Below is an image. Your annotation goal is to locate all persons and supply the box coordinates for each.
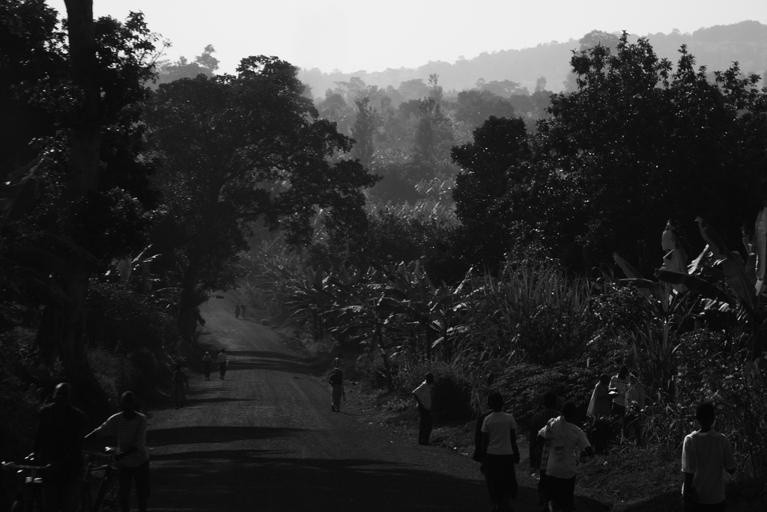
[35,382,153,511]
[327,365,346,413]
[200,348,229,381]
[474,391,520,511]
[680,401,738,512]
[172,364,189,409]
[530,400,595,512]
[587,365,645,455]
[410,370,436,447]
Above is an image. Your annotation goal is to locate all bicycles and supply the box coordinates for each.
[77,447,119,509]
[0,460,51,511]
